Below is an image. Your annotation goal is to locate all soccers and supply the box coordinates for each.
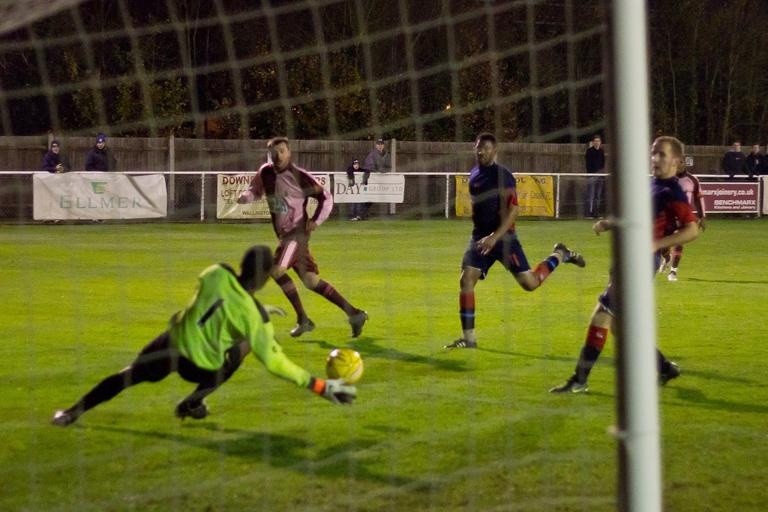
[326,349,363,384]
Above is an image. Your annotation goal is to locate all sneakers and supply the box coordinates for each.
[174,400,208,419]
[445,338,477,350]
[659,254,677,282]
[53,410,70,426]
[549,378,589,394]
[348,310,369,337]
[659,363,680,384]
[553,243,586,267]
[290,320,315,336]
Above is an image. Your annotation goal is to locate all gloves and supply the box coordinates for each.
[264,305,286,317]
[311,377,357,404]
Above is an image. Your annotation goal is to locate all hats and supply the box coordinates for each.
[96,135,106,143]
[376,138,385,144]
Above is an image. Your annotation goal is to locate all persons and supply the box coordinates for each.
[364,138,392,173]
[660,156,706,283]
[746,143,765,176]
[585,135,605,220]
[50,244,358,425]
[346,159,370,221]
[765,145,768,175]
[235,137,369,338]
[42,141,66,224]
[722,139,753,220]
[442,132,585,351]
[85,133,117,225]
[547,136,707,394]
